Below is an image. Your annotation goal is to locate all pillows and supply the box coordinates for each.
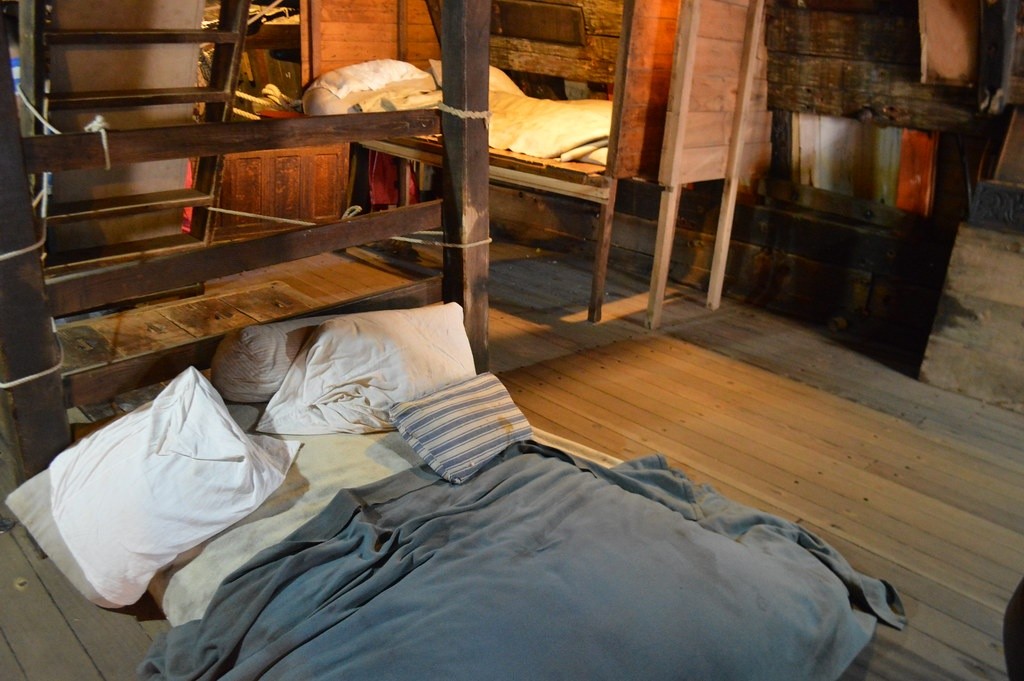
[427,58,526,97]
[252,302,477,435]
[3,364,304,612]
[305,58,429,100]
[299,87,442,119]
[386,372,534,484]
[211,301,444,403]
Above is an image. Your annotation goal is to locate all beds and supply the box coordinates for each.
[343,102,735,326]
[60,374,910,679]
